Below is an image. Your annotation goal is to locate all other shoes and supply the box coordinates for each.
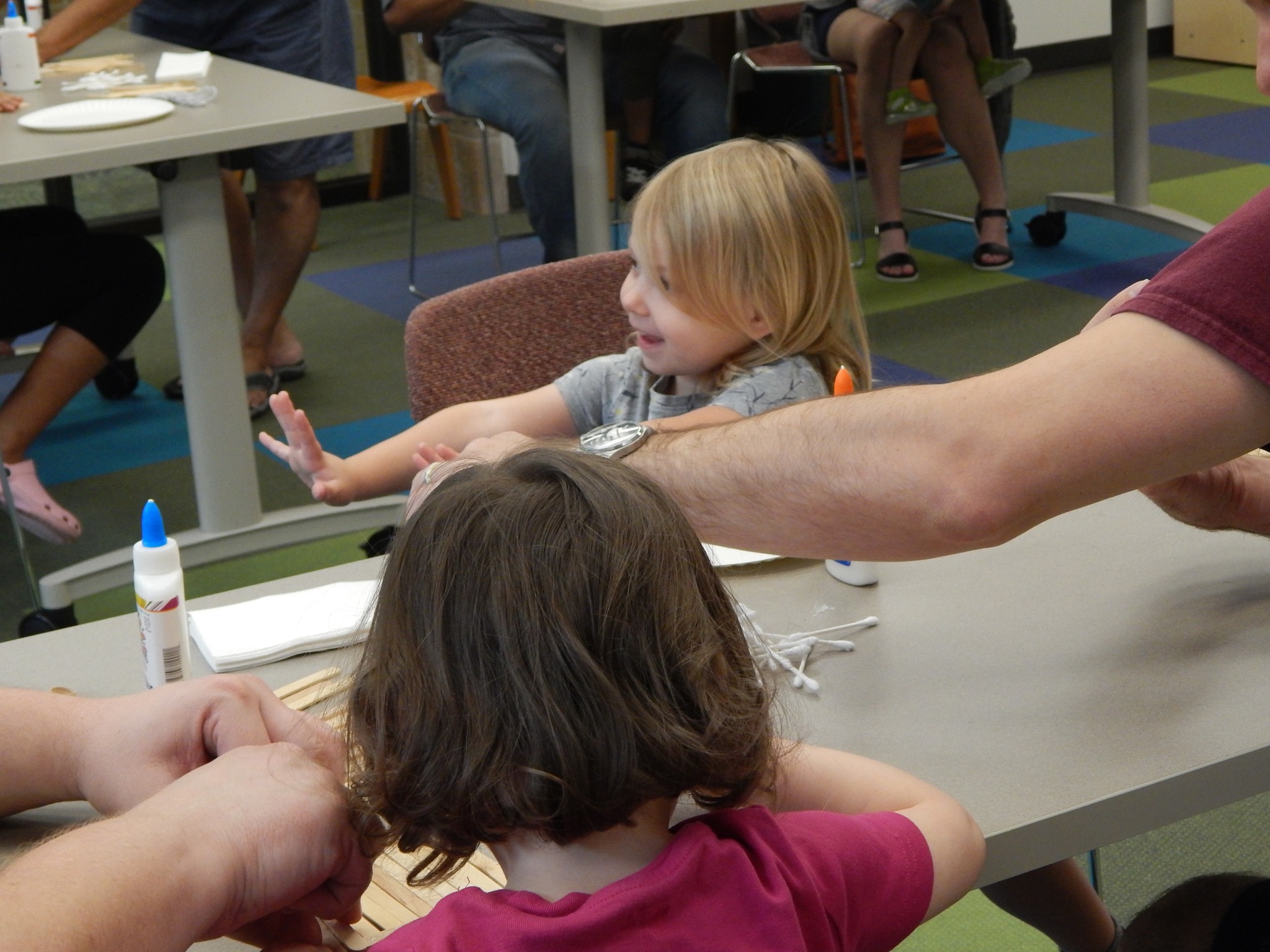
[885,87,939,125]
[978,56,1032,99]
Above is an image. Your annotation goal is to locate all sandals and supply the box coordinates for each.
[971,199,1015,272]
[871,215,920,282]
[0,458,82,545]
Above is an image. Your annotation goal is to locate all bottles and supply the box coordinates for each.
[0,0,43,93]
[133,498,187,691]
[824,364,879,585]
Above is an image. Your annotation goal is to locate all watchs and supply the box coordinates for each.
[577,421,659,462]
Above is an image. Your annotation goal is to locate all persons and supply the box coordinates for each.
[0,0,1031,541]
[338,451,986,952]
[258,139,1129,952]
[0,669,385,952]
[400,0,1270,952]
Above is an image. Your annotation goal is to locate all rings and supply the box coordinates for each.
[426,462,438,483]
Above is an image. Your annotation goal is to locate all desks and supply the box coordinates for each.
[2,488,1269,952]
[0,17,411,639]
[477,1,1219,268]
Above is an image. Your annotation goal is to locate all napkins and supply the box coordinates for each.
[154,51,213,82]
[188,579,384,672]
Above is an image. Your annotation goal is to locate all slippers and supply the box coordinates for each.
[244,370,281,422]
[272,359,307,381]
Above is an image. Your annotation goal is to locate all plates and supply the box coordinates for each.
[16,97,175,132]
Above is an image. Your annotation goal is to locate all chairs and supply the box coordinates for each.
[408,33,667,289]
[403,248,1101,896]
[726,0,1012,268]
